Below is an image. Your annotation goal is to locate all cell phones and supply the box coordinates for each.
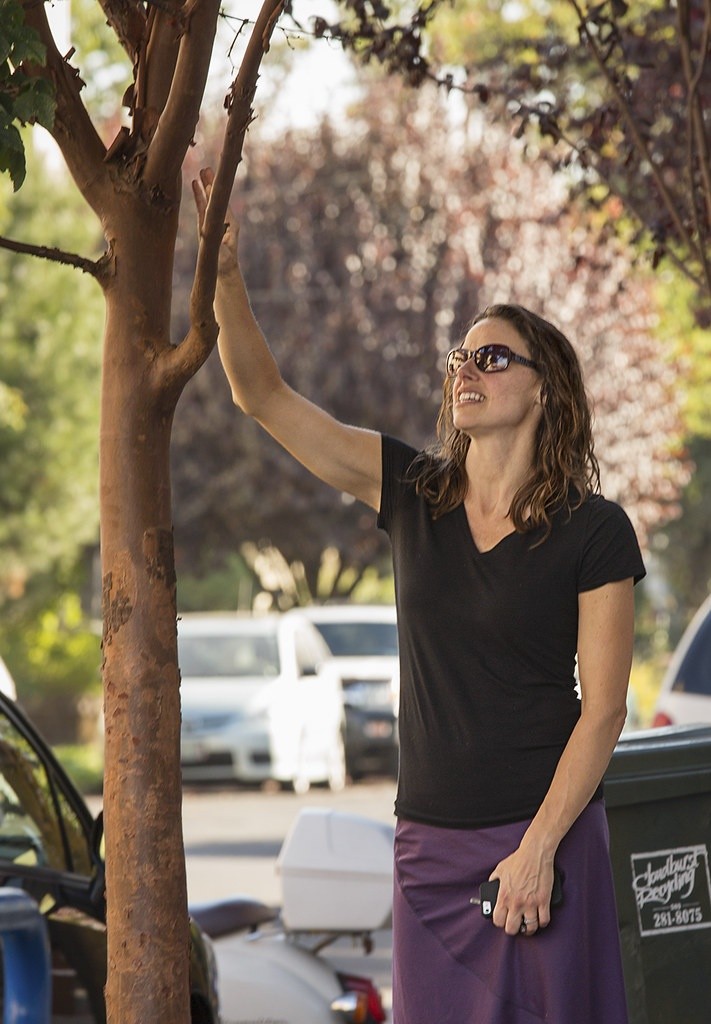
[479,873,565,916]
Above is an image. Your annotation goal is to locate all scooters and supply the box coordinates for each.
[186,801,395,1024]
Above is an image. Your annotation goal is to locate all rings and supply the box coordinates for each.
[524,918,538,924]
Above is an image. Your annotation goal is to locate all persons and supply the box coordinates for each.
[191,168,646,1024]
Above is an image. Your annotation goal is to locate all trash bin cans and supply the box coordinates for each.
[602,724,711,1023]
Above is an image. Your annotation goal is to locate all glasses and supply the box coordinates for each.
[446,343,547,378]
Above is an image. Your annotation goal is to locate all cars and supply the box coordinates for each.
[2,686,223,1024]
[94,610,349,795]
[651,590,711,732]
[295,602,402,785]
